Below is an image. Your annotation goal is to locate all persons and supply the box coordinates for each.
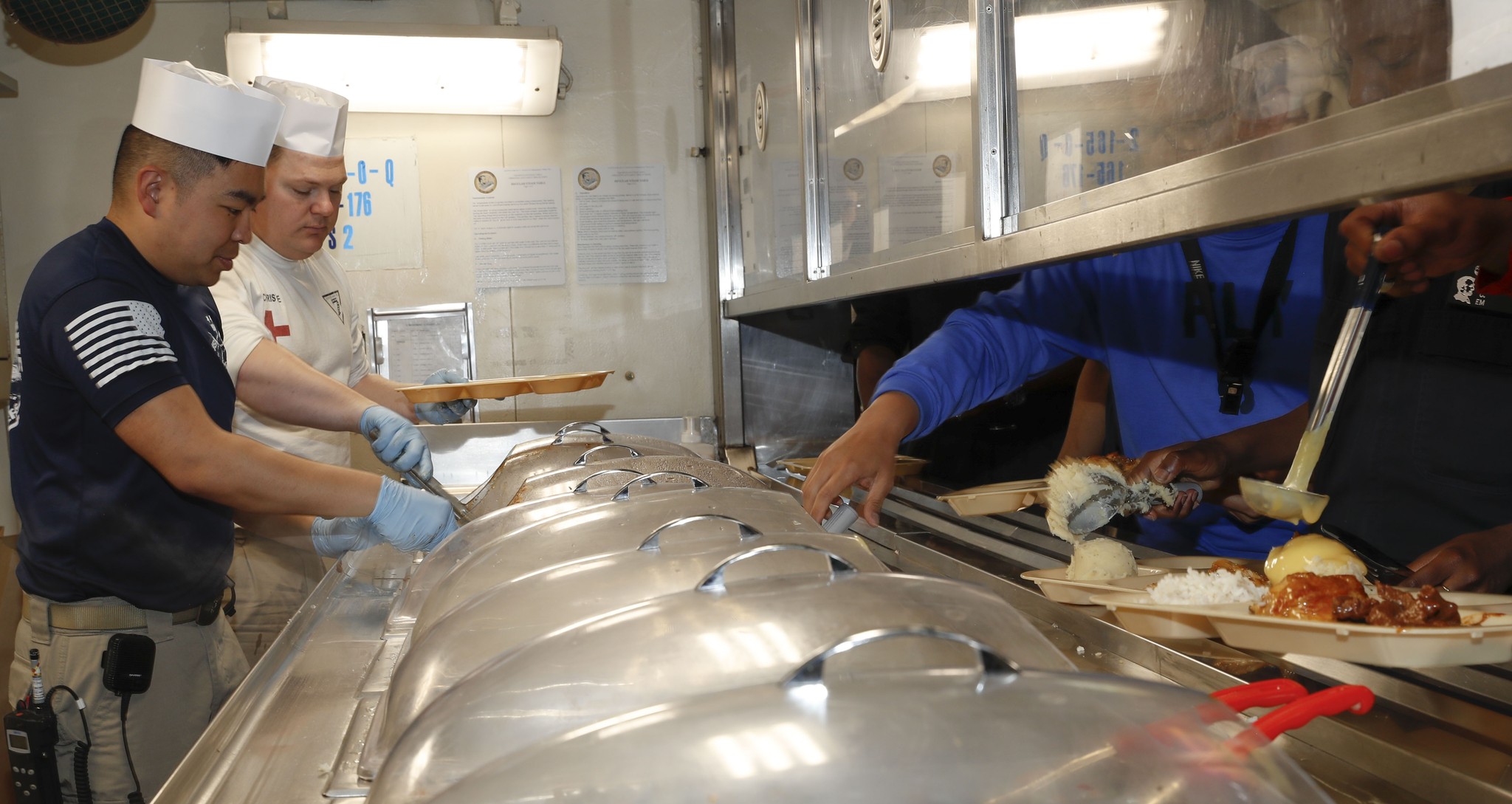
[5,57,461,804]
[208,76,504,672]
[800,0,1512,726]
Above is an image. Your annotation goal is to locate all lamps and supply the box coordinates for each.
[222,0,566,119]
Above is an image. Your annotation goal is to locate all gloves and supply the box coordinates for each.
[365,475,460,555]
[312,517,385,559]
[360,405,434,489]
[417,369,479,426]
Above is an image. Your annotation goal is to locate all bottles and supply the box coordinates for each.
[681,416,701,443]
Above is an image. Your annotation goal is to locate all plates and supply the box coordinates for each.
[394,370,616,405]
[937,479,1049,517]
[779,453,931,480]
[1020,556,1267,606]
[1089,585,1512,669]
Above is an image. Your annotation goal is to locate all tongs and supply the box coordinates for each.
[369,428,470,519]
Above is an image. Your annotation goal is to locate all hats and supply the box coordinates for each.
[253,75,349,158]
[131,57,286,167]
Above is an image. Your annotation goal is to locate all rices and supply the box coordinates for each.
[1145,567,1271,606]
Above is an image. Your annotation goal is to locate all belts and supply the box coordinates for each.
[20,591,223,629]
